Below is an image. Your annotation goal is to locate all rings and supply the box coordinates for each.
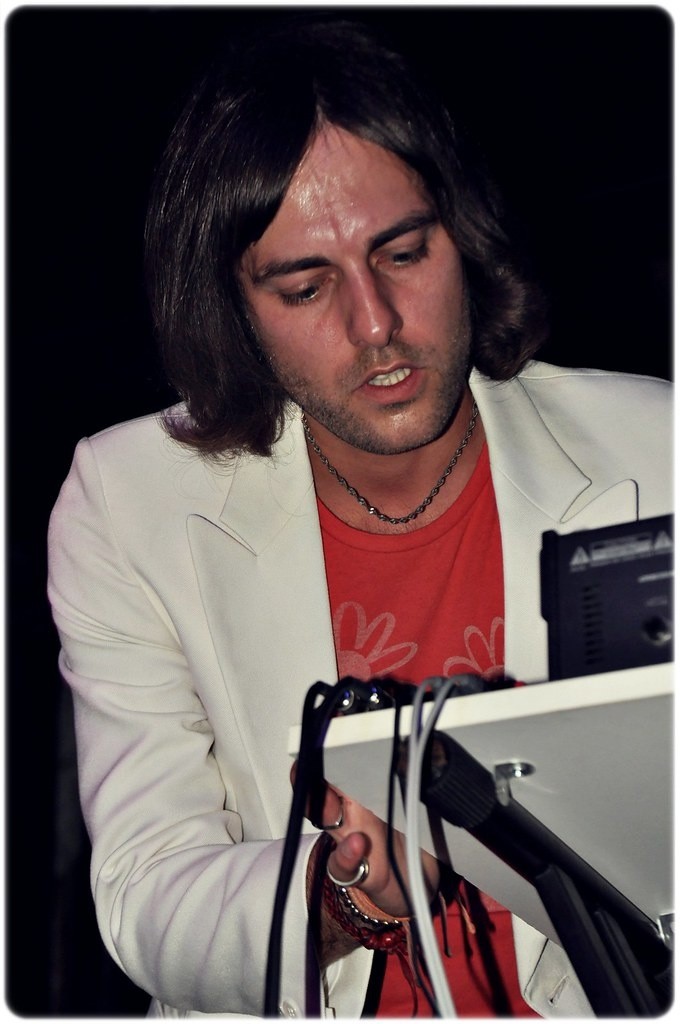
[310,805,344,831]
[326,857,370,888]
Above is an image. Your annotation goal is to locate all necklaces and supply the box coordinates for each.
[300,399,480,524]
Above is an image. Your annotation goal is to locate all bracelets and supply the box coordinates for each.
[321,837,476,1018]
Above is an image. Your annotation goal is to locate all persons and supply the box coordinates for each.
[48,25,675,1019]
